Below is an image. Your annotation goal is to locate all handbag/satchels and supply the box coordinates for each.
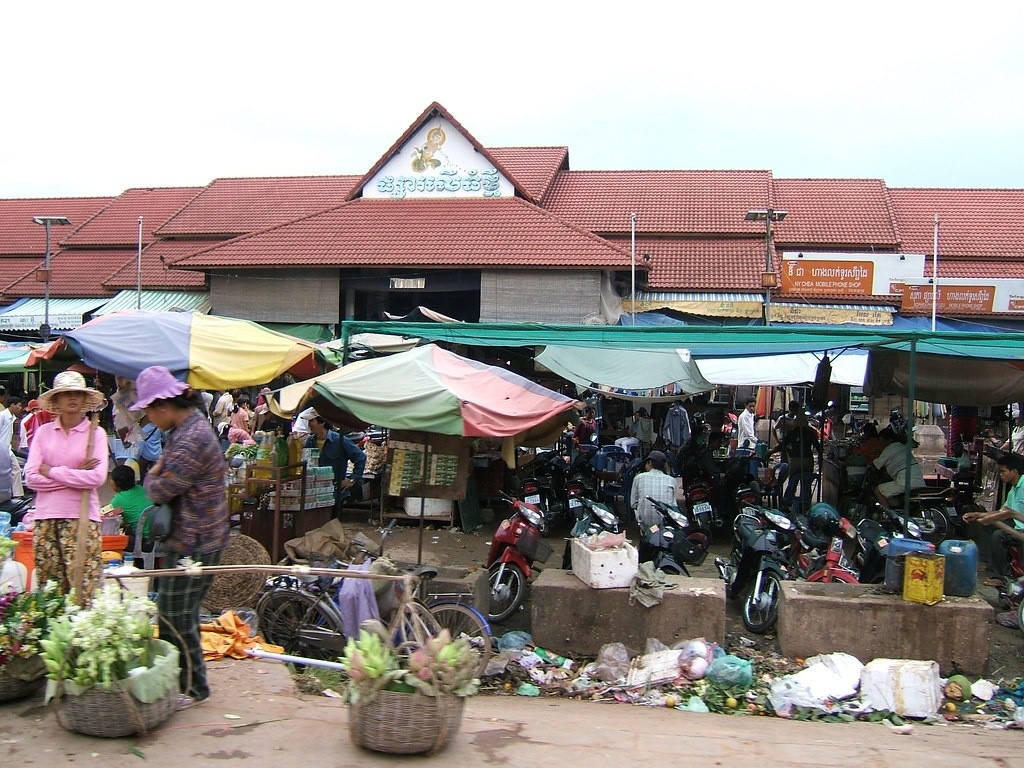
[148,502,172,540]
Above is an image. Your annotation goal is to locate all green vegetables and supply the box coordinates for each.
[223,443,260,459]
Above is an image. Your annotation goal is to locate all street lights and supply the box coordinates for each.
[742,209,789,328]
[32,215,73,342]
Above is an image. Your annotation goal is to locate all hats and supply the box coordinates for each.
[640,451,667,462]
[25,400,38,412]
[300,407,319,420]
[218,422,229,436]
[38,371,104,414]
[261,387,271,395]
[794,415,809,426]
[258,403,269,414]
[129,366,190,413]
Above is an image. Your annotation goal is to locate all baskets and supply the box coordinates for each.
[200,533,272,614]
[0,676,45,704]
[56,638,179,737]
[672,532,709,566]
[517,528,553,564]
[349,693,465,754]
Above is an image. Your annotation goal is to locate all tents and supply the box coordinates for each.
[340,319,1024,539]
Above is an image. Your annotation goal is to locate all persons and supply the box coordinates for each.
[630,451,677,532]
[772,400,800,483]
[628,411,644,437]
[110,465,155,552]
[572,406,597,454]
[0,387,40,505]
[128,364,229,712]
[25,370,109,609]
[769,412,821,513]
[737,396,764,450]
[852,421,883,460]
[199,387,279,445]
[870,428,927,508]
[962,455,1024,586]
[139,423,167,486]
[676,425,725,479]
[1000,410,1024,455]
[604,402,621,429]
[292,406,367,521]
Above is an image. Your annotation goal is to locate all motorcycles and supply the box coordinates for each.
[567,450,604,520]
[640,495,715,578]
[783,500,865,584]
[858,500,920,584]
[847,462,955,546]
[720,409,739,438]
[504,447,570,535]
[488,487,555,622]
[654,409,724,540]
[805,399,838,452]
[690,410,713,450]
[843,413,881,440]
[0,497,35,527]
[344,431,389,450]
[887,406,910,444]
[722,437,765,511]
[562,490,624,567]
[715,480,808,633]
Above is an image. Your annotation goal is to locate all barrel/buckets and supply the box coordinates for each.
[885,537,980,606]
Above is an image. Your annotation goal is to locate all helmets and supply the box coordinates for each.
[805,502,842,538]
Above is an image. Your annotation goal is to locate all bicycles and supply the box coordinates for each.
[257,521,492,689]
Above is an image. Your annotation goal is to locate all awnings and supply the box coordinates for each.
[0,290,210,331]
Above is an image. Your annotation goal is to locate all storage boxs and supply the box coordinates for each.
[570,538,638,590]
[402,495,452,518]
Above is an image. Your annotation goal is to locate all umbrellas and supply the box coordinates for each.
[25,306,344,391]
[0,341,43,373]
[256,342,587,565]
[142,610,351,675]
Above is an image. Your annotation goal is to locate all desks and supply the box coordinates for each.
[934,463,959,488]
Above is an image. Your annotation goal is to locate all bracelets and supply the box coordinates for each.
[349,479,354,483]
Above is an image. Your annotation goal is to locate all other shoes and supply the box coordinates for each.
[979,561,989,572]
[983,577,1004,586]
[995,610,1020,628]
[179,694,210,710]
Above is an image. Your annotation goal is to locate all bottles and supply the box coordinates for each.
[1,556,151,606]
[253,428,303,483]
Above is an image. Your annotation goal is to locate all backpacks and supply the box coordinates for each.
[682,449,710,481]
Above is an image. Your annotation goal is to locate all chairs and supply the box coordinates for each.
[575,442,642,520]
[124,504,164,592]
